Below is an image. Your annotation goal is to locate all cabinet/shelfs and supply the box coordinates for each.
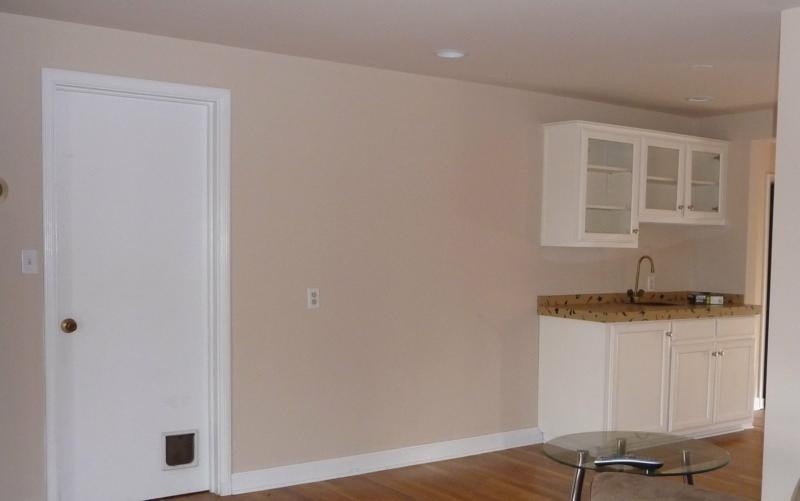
[641,129,726,226]
[670,316,756,435]
[538,119,642,249]
[537,317,671,453]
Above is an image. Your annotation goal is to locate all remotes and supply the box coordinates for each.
[594,455,665,470]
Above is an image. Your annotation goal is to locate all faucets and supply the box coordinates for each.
[635,254,654,296]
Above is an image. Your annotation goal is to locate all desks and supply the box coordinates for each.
[540,430,733,500]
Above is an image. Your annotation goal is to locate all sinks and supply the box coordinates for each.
[631,303,677,306]
